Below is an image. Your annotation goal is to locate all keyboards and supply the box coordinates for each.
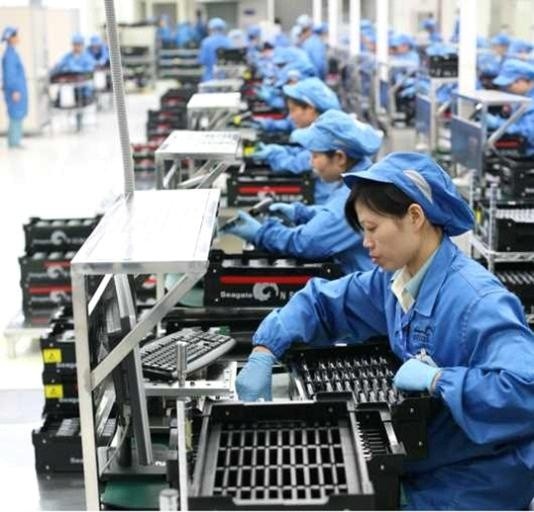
[133,328,236,382]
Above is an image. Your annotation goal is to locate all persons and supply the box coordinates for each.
[156,13,173,47]
[0,25,30,149]
[449,8,463,45]
[476,53,500,89]
[213,106,385,274]
[290,11,311,46]
[477,37,488,50]
[418,12,444,56]
[360,18,374,96]
[191,9,208,46]
[195,18,235,81]
[247,75,339,134]
[241,22,264,61]
[387,24,430,120]
[175,20,191,49]
[87,35,110,69]
[256,40,318,81]
[489,35,510,54]
[292,25,326,81]
[234,148,534,507]
[484,59,534,159]
[229,27,247,45]
[48,33,97,133]
[509,40,534,59]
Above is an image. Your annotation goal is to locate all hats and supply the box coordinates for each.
[311,21,329,35]
[269,47,312,67]
[71,29,84,46]
[246,25,263,43]
[502,34,531,55]
[489,56,532,93]
[280,73,339,116]
[0,20,19,44]
[475,48,502,78]
[282,63,320,84]
[337,149,479,239]
[89,36,101,48]
[384,30,415,54]
[205,15,226,34]
[287,107,388,161]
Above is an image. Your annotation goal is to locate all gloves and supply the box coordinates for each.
[389,356,444,398]
[219,212,263,249]
[252,141,277,163]
[232,350,277,409]
[266,199,297,229]
